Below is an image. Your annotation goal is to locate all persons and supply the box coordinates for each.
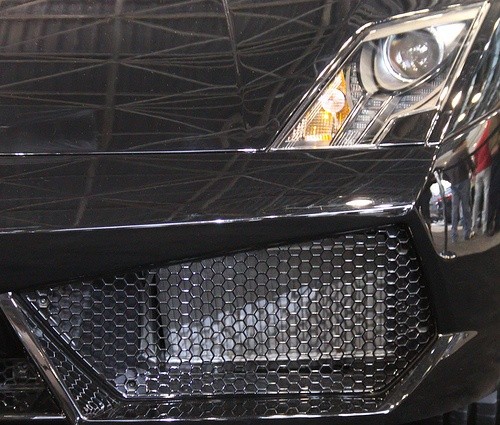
[443,138,476,244]
[471,132,499,236]
